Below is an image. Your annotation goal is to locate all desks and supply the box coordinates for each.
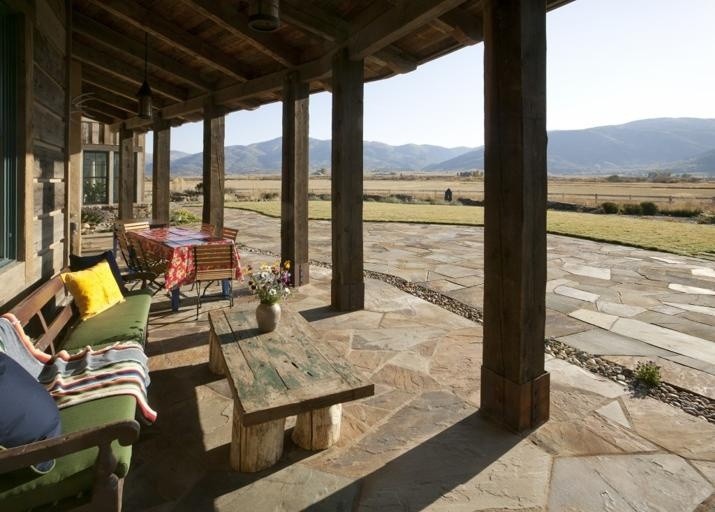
[207,305,375,474]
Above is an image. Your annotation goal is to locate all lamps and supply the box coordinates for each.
[136,32,153,119]
[247,0,281,34]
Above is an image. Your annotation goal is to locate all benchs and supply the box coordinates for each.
[0,266,157,512]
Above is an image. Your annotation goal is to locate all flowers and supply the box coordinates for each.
[243,256,297,305]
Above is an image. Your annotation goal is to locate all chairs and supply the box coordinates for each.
[114,220,239,320]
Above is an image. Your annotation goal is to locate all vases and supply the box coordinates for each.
[255,304,281,332]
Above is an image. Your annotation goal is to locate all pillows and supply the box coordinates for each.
[0,351,61,474]
[59,249,128,323]
